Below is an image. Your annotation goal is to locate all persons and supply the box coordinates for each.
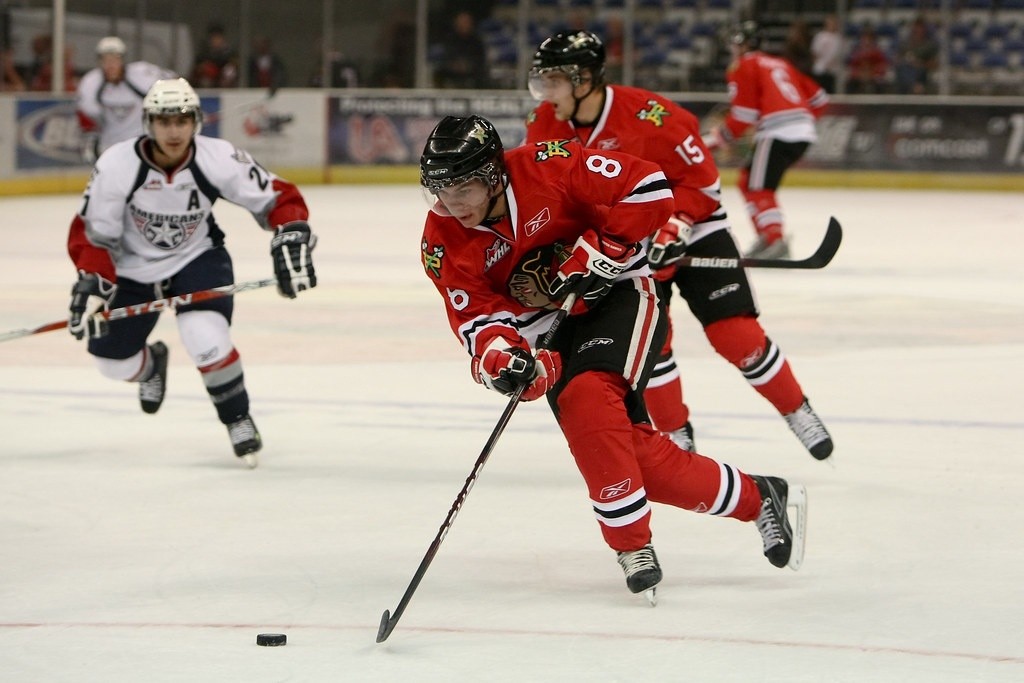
[0,34,77,91]
[186,26,294,88]
[636,4,722,117]
[526,30,833,461]
[700,20,830,258]
[603,18,641,85]
[67,77,318,457]
[311,37,346,88]
[787,15,939,95]
[432,9,486,89]
[421,113,794,596]
[74,36,180,166]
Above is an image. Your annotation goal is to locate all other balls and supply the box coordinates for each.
[256,633,287,647]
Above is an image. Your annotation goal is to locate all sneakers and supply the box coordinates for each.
[617,542,663,607]
[226,414,263,467]
[139,341,168,414]
[739,226,790,260]
[783,395,834,461]
[747,474,808,571]
[662,420,696,455]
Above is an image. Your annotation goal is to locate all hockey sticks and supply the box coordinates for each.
[674,216,843,270]
[0,277,279,342]
[375,291,576,644]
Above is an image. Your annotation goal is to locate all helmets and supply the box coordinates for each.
[95,36,127,55]
[728,21,764,52]
[143,77,200,116]
[419,115,505,193]
[527,28,607,85]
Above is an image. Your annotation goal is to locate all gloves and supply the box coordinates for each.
[80,131,99,165]
[648,222,679,282]
[68,267,118,341]
[471,325,562,402]
[270,221,317,299]
[547,227,637,316]
